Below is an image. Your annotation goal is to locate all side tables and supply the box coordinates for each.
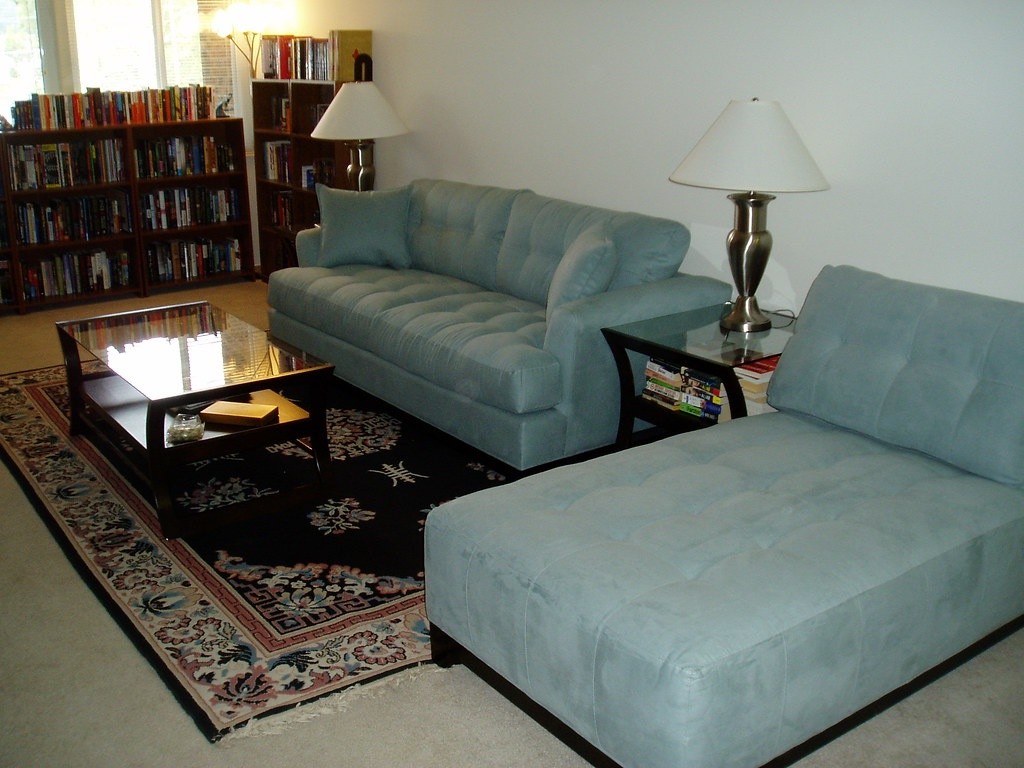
[601,305,797,455]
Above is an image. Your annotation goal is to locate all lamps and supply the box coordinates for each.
[211,0,298,79]
[668,100,832,331]
[309,82,406,190]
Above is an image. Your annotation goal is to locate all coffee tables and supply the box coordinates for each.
[54,301,335,542]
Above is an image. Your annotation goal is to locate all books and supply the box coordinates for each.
[185,393,245,414]
[4,83,246,303]
[63,303,231,354]
[199,400,279,428]
[262,94,332,231]
[639,354,782,424]
[260,29,373,83]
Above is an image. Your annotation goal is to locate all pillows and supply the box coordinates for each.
[546,219,616,326]
[315,183,409,270]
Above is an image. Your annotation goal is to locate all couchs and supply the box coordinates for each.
[268,177,732,478]
[423,264,1024,768]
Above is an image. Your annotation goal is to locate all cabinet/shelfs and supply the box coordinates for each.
[3,117,142,314]
[130,118,256,299]
[252,79,350,283]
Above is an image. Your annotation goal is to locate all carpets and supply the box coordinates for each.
[0,330,520,747]
